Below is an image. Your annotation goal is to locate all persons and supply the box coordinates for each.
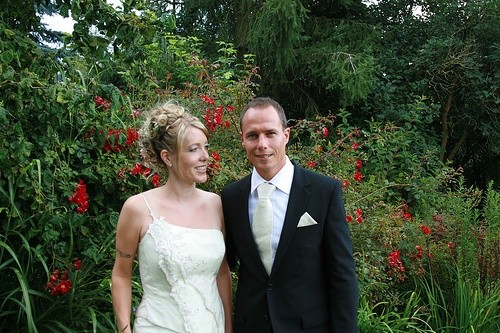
[218,98,359,332]
[111,100,233,333]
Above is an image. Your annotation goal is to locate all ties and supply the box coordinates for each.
[251,183,277,276]
